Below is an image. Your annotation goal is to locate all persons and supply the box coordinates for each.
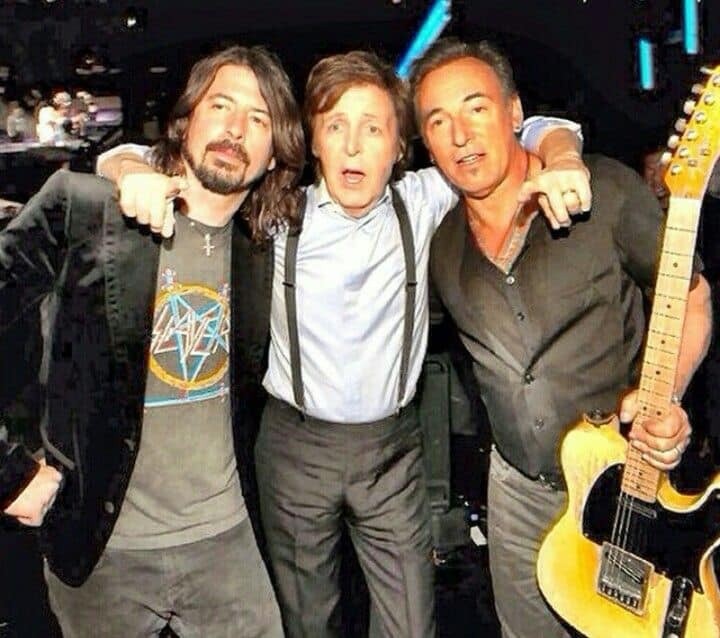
[0,42,720,638]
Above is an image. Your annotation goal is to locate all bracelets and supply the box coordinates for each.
[672,393,683,406]
[550,154,584,167]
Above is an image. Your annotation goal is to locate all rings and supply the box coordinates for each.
[562,189,576,196]
[675,446,681,457]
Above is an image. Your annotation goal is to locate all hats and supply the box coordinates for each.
[53,92,72,106]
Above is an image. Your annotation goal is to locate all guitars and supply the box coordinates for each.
[534,64,720,638]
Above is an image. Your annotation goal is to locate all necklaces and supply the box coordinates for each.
[493,147,531,266]
[177,210,234,258]
